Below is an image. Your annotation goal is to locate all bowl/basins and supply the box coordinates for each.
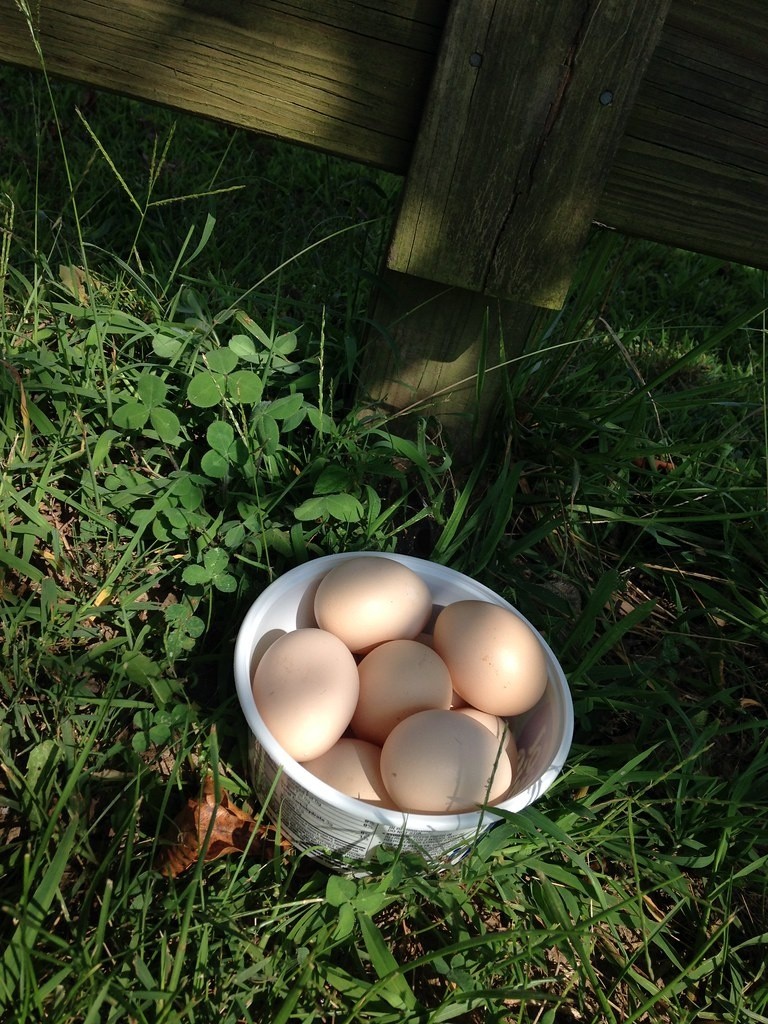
[235,550,576,874]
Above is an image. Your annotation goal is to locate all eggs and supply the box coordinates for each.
[251,556,548,814]
